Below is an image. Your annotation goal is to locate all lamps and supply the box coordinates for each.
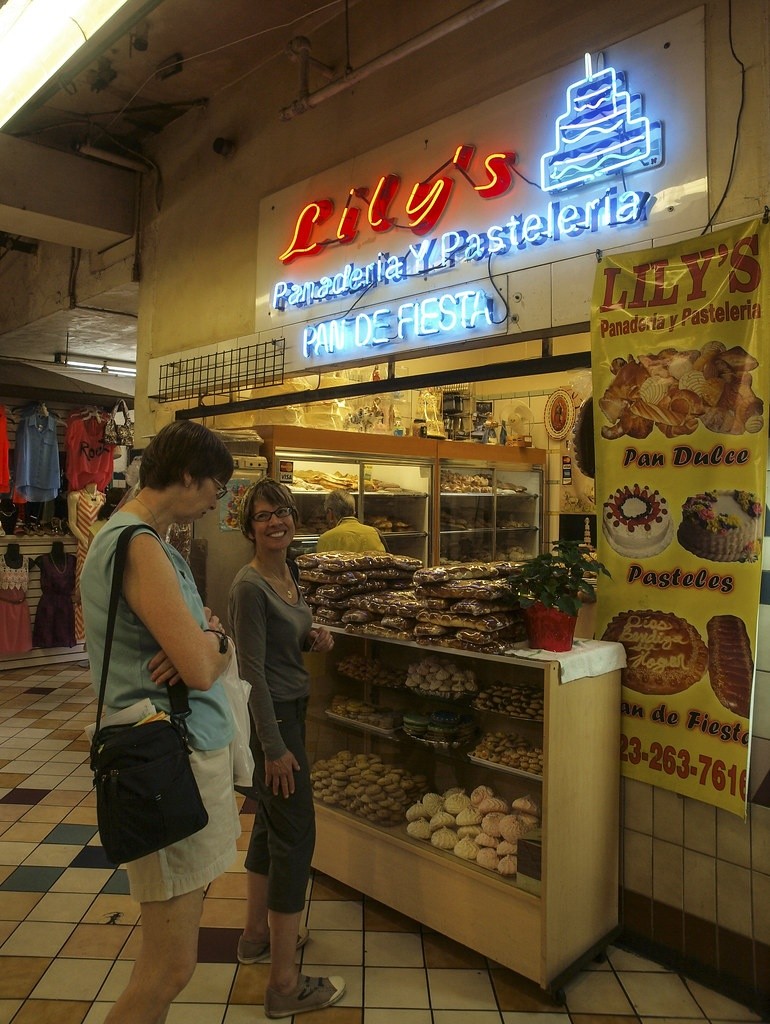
[54,353,136,378]
[277,1,515,125]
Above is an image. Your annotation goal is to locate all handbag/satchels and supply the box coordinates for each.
[91,720,209,864]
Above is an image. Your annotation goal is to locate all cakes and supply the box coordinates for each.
[677,489,762,562]
[602,483,675,558]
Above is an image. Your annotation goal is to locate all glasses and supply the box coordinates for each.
[210,476,228,499]
[250,507,293,522]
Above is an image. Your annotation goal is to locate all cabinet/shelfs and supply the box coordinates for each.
[250,425,437,572]
[306,611,626,988]
[432,438,548,569]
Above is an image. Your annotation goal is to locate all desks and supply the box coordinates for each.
[574,577,598,639]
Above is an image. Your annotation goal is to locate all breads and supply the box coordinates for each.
[598,340,764,439]
[600,608,709,694]
[292,468,543,875]
[706,614,754,718]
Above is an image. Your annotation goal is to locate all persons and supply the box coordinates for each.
[68,482,108,642]
[86,487,125,552]
[79,420,244,1023]
[224,475,346,1020]
[314,488,387,555]
[1,543,38,653]
[31,542,79,649]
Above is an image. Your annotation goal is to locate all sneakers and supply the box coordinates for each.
[238,925,309,965]
[265,973,348,1019]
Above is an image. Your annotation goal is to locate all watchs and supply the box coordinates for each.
[204,628,229,654]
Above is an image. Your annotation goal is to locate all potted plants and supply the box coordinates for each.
[509,538,612,652]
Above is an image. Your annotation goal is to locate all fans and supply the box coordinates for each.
[499,400,535,447]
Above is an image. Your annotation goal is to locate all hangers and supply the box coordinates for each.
[11,400,114,422]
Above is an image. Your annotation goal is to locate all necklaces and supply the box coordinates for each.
[257,555,293,600]
[133,498,161,537]
